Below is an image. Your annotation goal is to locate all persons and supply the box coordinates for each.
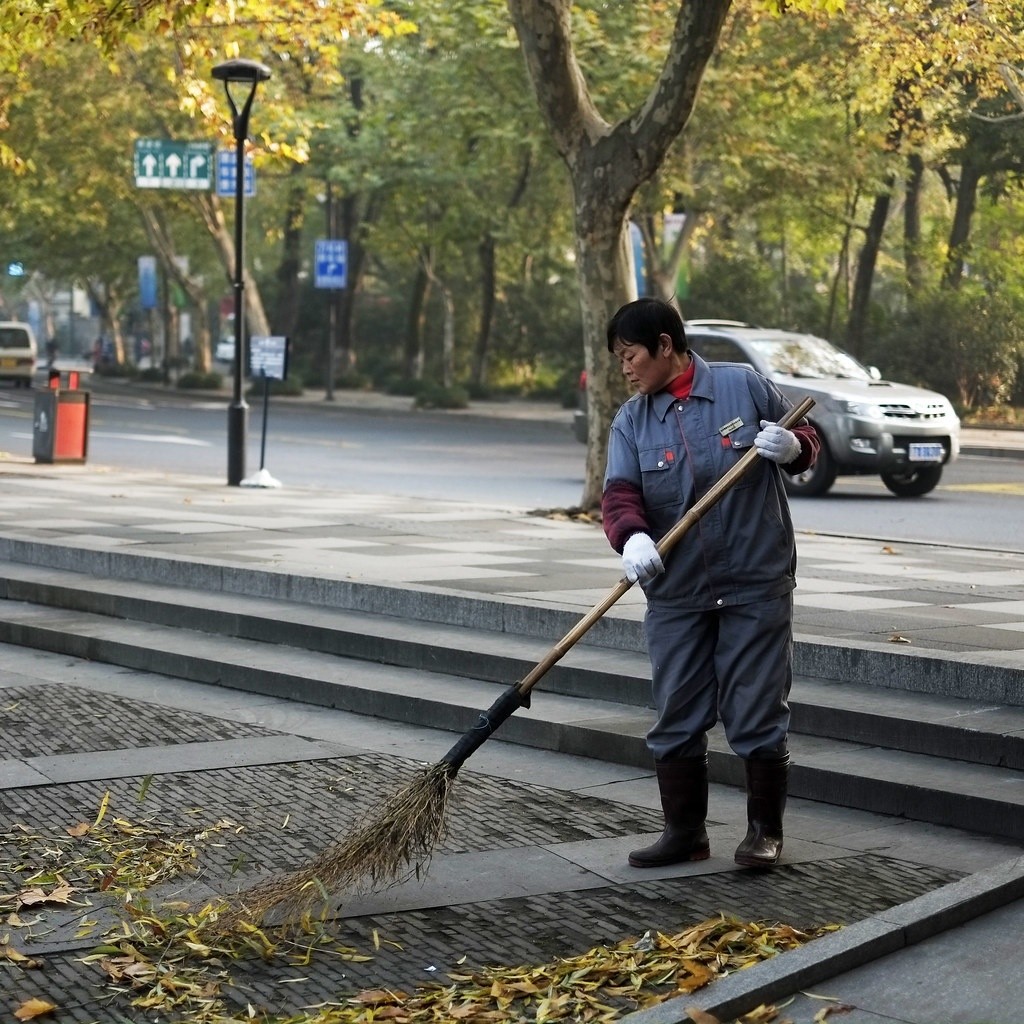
[602,296,820,871]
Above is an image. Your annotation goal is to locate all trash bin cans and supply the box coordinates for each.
[31,360,95,465]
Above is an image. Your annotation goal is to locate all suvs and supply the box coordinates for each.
[684,316,964,500]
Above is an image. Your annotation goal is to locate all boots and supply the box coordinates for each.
[734,749,790,868]
[627,752,712,867]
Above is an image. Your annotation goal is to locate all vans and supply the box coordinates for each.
[0,319,39,387]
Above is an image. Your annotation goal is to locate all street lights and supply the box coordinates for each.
[210,60,273,489]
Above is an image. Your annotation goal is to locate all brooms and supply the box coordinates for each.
[215,398,820,939]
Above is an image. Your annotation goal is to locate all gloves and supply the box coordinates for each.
[621,532,664,586]
[754,419,802,464]
